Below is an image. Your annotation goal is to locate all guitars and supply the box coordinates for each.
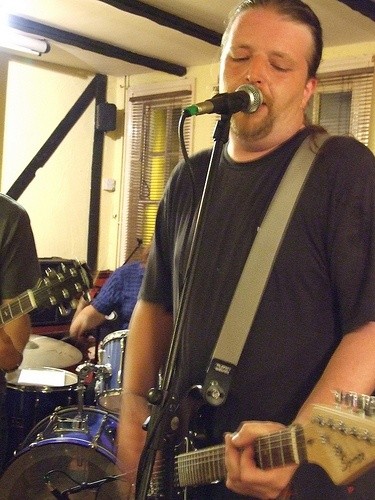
[0,259,98,334]
[130,386,373,499]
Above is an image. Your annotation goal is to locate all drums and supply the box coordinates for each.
[1,407,134,500]
[92,328,141,412]
[2,364,80,451]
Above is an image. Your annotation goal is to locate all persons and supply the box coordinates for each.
[1,194,39,471]
[69,254,149,337]
[115,0,374,500]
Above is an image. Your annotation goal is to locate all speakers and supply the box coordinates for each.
[96,103,116,131]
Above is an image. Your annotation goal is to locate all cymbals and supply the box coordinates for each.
[11,333,84,369]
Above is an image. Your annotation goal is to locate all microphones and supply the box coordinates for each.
[185,84,265,119]
